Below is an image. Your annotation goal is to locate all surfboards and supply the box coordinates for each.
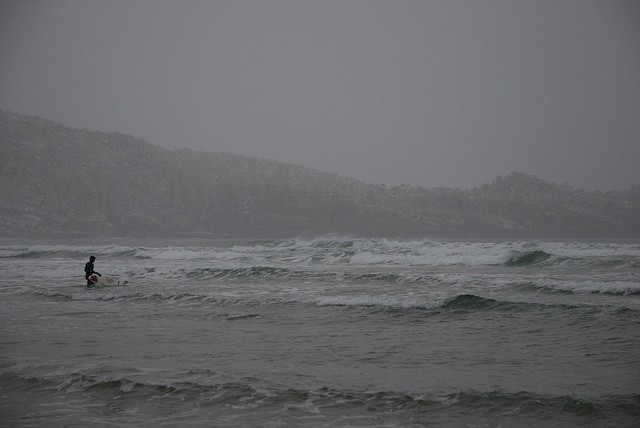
[87,274,130,287]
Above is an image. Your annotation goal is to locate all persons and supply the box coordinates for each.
[83,255,102,286]
